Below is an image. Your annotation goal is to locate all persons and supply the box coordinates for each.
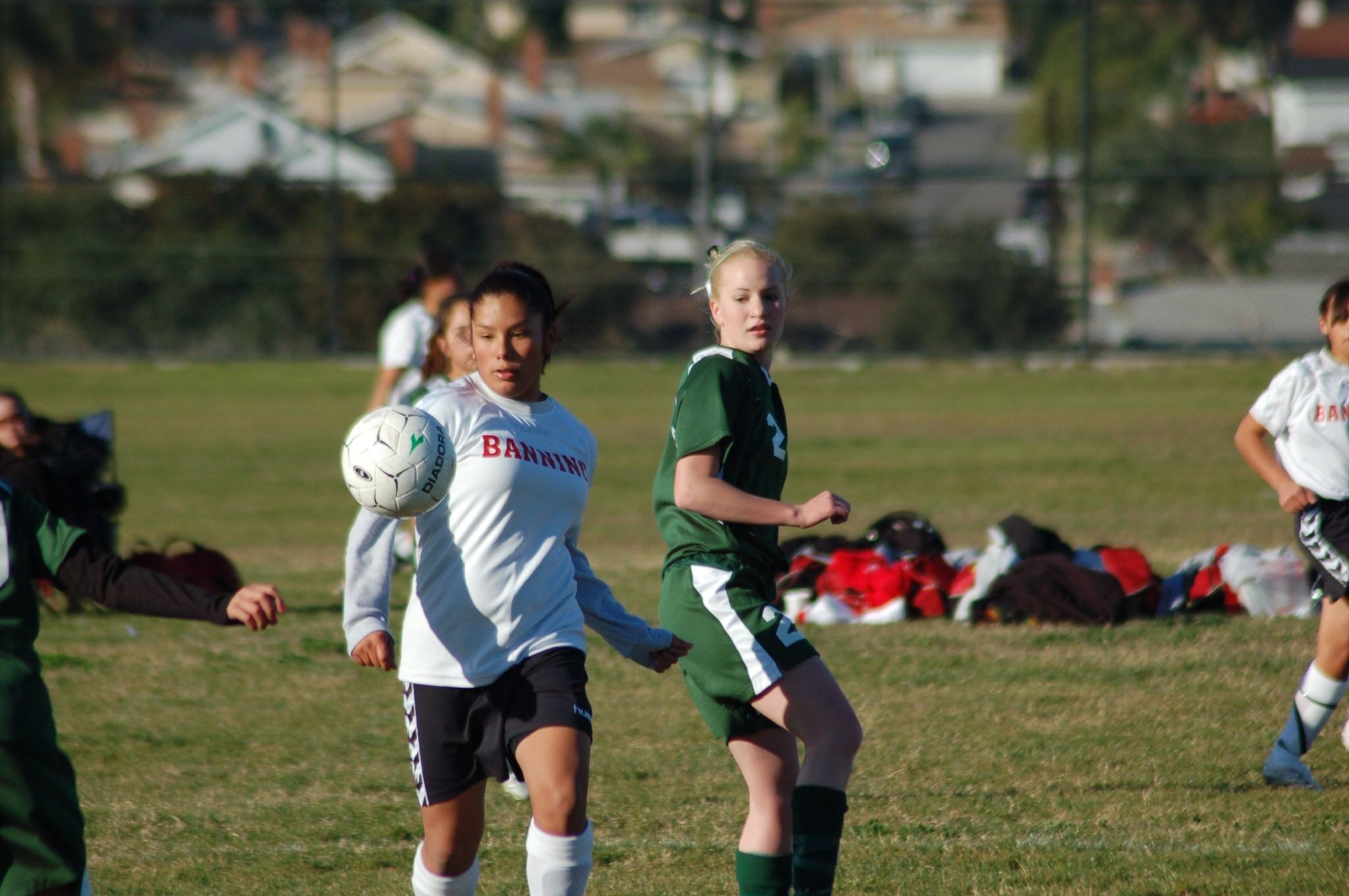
[651,238,864,896]
[1235,282,1348,792]
[0,395,287,896]
[368,255,534,802]
[344,265,694,896]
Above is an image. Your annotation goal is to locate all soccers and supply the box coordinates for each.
[340,406,457,521]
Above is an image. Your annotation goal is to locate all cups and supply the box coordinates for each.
[784,587,811,623]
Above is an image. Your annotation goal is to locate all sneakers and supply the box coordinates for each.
[1263,753,1327,794]
